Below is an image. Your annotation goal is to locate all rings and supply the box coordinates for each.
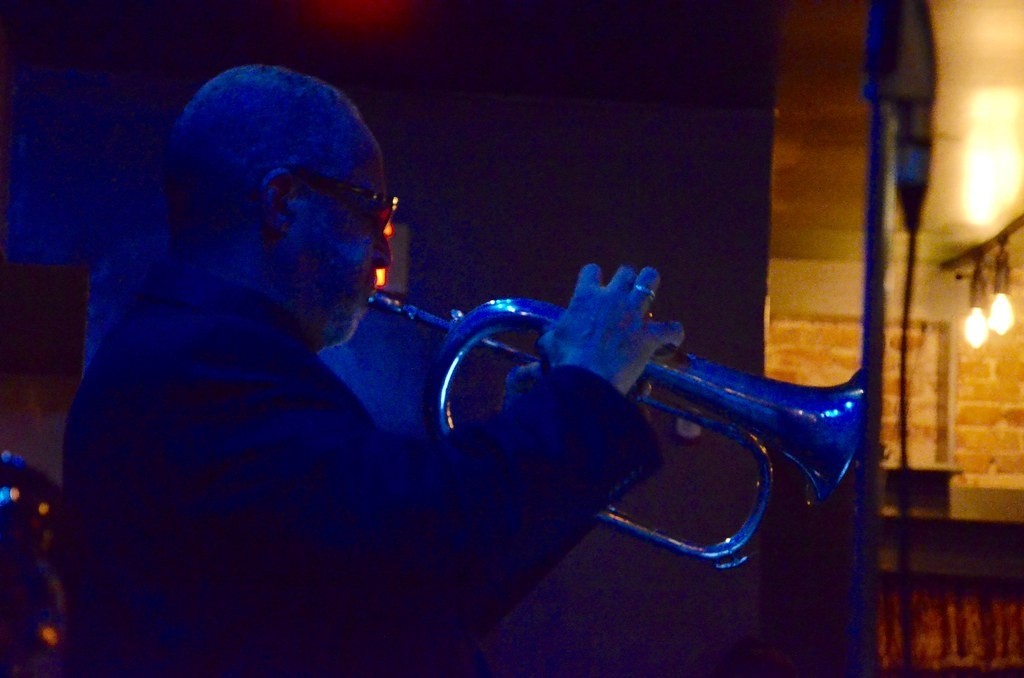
[634,283,656,298]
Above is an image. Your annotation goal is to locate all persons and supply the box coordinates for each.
[51,66,687,678]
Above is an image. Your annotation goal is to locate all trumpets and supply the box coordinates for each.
[366,291,871,571]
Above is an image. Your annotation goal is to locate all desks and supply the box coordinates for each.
[876,487,1024,678]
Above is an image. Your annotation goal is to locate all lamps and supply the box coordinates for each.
[963,247,989,347]
[988,233,1015,334]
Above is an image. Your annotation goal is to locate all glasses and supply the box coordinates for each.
[252,167,398,235]
